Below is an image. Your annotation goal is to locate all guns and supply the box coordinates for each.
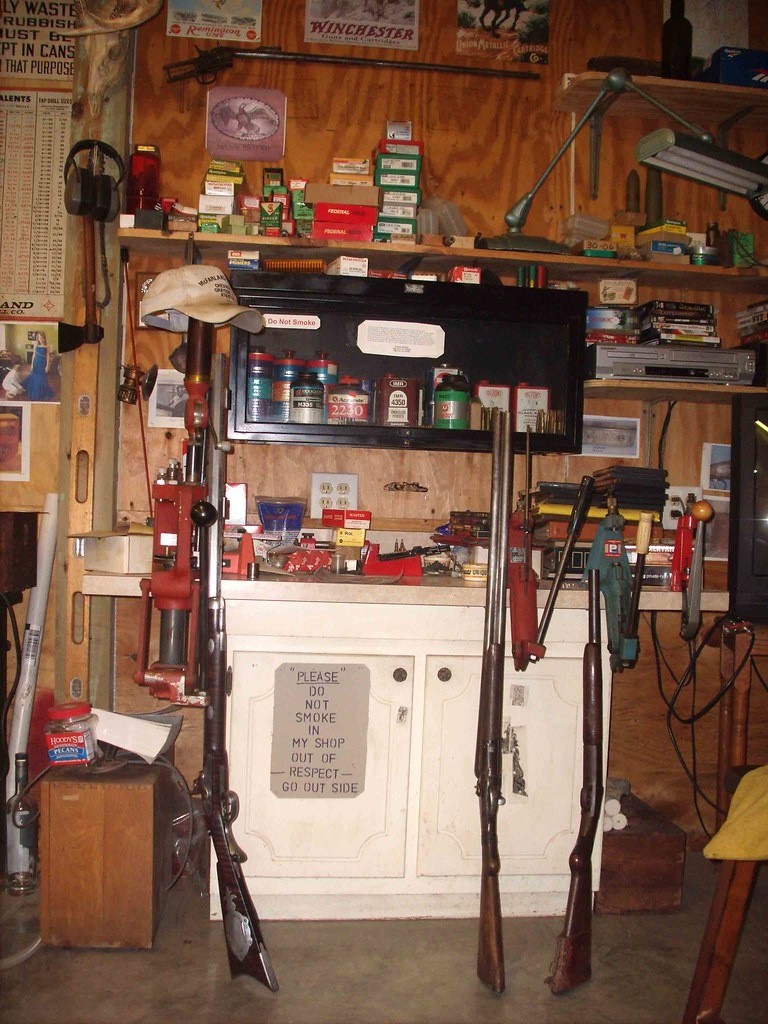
[472,407,513,998]
[543,567,605,996]
[190,355,280,995]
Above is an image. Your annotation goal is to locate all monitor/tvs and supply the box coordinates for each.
[727,393,768,621]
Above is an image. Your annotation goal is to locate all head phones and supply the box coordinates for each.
[63,138,126,223]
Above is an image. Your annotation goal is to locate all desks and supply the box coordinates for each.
[719,623,768,863]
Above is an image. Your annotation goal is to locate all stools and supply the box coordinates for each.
[684,765,768,1024]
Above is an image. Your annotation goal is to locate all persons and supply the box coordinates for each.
[2,331,49,400]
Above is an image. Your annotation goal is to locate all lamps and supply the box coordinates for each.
[475,67,768,254]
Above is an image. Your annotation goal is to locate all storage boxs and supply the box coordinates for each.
[304,158,382,243]
[569,213,691,264]
[372,120,424,245]
[329,528,367,562]
[85,534,154,573]
[712,47,768,90]
[323,510,372,529]
[41,763,172,951]
[595,791,687,912]
[198,159,245,233]
[223,179,314,238]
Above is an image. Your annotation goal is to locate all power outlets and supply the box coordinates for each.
[311,473,358,519]
[662,485,702,530]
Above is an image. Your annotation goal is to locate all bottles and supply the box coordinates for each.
[289,373,324,424]
[433,374,470,430]
[327,376,370,426]
[706,221,721,247]
[661,0,693,79]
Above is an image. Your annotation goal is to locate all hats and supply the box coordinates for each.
[139,265,265,332]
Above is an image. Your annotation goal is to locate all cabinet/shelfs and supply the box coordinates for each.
[208,572,730,929]
[118,72,768,404]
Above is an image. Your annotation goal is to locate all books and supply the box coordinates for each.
[90,704,183,766]
